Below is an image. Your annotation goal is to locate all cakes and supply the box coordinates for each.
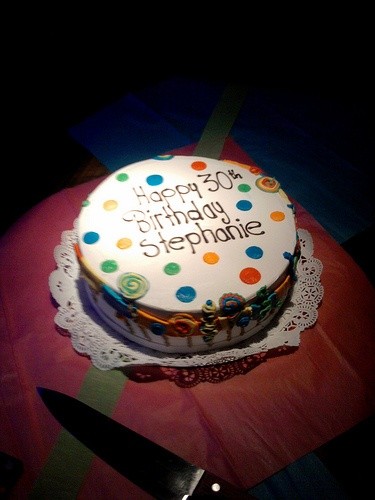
[75,153,301,355]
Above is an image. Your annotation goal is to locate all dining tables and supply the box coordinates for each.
[0,136,374,500]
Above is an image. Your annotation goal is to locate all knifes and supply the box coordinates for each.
[40,385,240,500]
